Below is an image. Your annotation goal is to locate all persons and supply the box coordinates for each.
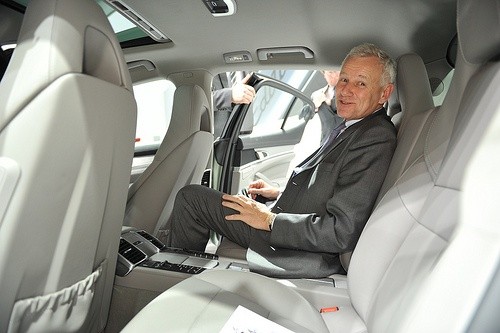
[212,72,256,139]
[168,42,399,279]
[310,68,343,148]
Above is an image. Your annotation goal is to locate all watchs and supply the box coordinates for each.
[267,214,277,231]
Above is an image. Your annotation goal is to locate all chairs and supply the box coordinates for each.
[123,84,213,243]
[1,0,137,333]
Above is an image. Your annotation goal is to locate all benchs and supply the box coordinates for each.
[121,0,500,333]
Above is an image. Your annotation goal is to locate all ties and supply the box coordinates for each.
[286,119,347,181]
[229,71,238,87]
